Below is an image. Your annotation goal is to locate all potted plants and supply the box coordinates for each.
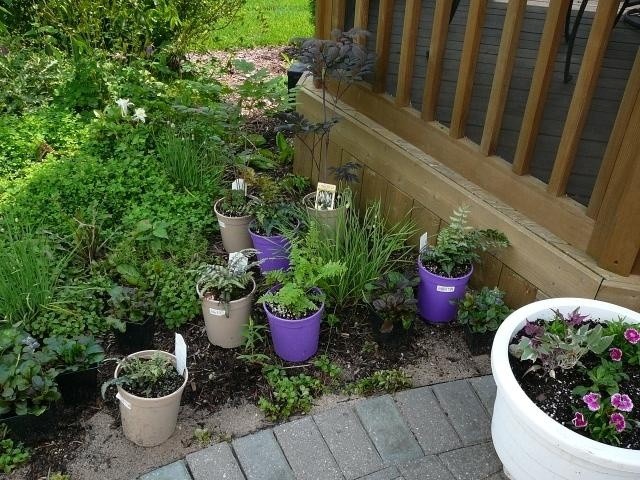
[304,179,354,244]
[213,180,264,254]
[262,248,325,364]
[248,181,306,277]
[112,349,189,447]
[454,285,510,354]
[418,200,509,323]
[193,248,262,349]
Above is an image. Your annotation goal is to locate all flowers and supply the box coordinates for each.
[512,305,639,448]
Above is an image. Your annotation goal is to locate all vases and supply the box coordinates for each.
[489,297,639,480]
